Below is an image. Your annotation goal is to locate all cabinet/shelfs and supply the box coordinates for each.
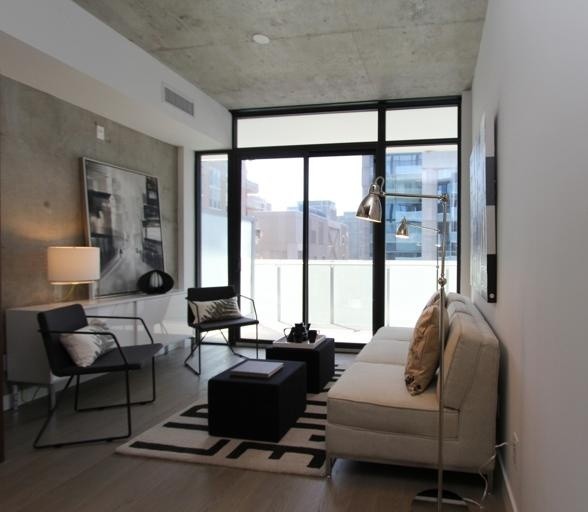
[5,289,194,411]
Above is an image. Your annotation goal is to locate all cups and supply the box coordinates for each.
[308,330,317,342]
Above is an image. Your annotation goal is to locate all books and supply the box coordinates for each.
[231,360,284,378]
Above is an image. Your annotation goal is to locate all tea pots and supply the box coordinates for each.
[283,327,305,342]
[294,323,311,338]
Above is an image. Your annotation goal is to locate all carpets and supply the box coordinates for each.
[115,364,349,475]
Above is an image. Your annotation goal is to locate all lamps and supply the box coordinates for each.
[355,178,468,512]
[393,216,441,293]
[46,246,101,301]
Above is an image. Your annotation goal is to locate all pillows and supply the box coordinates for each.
[406,307,449,394]
[422,287,447,309]
[188,297,243,323]
[61,319,115,366]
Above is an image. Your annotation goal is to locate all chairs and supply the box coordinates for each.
[185,286,258,373]
[33,302,162,449]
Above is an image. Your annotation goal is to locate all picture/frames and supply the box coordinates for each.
[81,158,164,302]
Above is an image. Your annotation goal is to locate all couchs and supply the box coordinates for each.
[324,293,500,494]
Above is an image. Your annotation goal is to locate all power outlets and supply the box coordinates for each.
[511,434,520,463]
